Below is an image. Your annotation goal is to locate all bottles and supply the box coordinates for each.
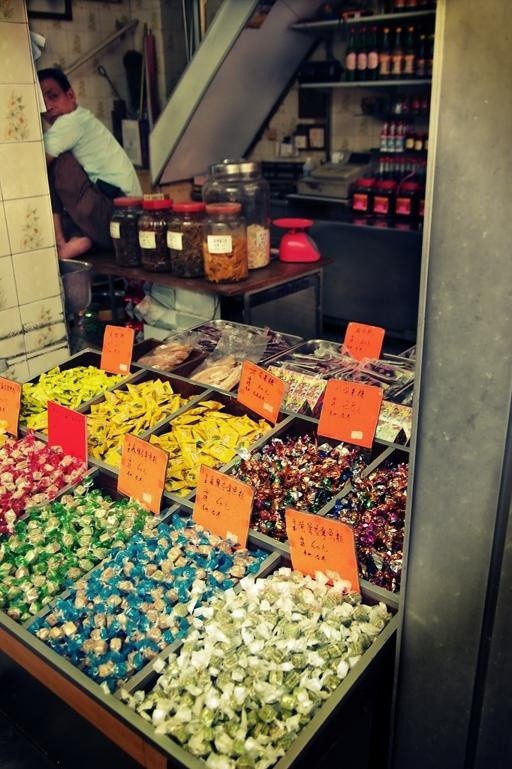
[374,159,425,185]
[303,157,315,177]
[347,25,433,77]
[201,159,272,268]
[378,96,428,154]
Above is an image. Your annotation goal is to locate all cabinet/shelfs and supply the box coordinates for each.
[290,7,436,94]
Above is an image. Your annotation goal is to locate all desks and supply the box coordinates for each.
[83,246,336,342]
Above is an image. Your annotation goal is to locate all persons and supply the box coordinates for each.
[33,68,144,257]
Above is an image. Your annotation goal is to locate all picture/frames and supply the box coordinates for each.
[25,0,74,21]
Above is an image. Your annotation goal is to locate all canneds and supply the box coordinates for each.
[351,177,419,223]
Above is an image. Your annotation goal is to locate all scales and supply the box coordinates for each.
[272,218,321,262]
